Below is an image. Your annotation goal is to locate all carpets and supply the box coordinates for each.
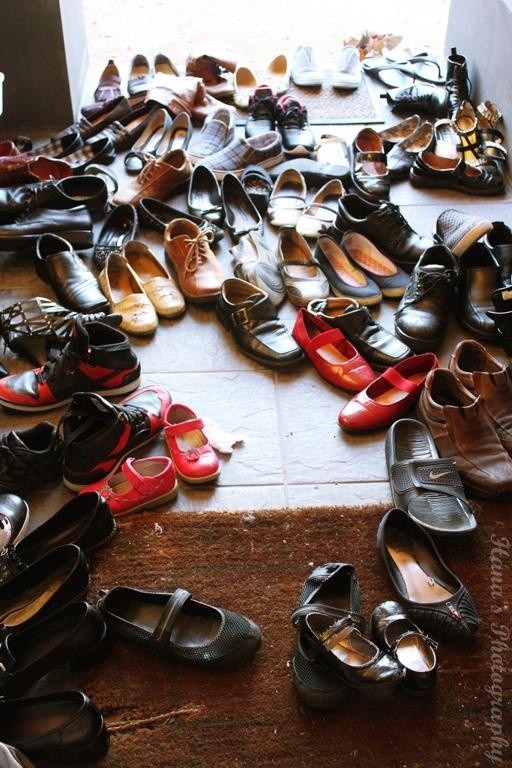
[231,62,386,128]
[24,494,511,768]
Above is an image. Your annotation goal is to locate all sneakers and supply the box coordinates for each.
[1,320,262,766]
[291,297,512,709]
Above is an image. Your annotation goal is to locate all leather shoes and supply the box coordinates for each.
[218,276,304,368]
[0,45,511,338]
